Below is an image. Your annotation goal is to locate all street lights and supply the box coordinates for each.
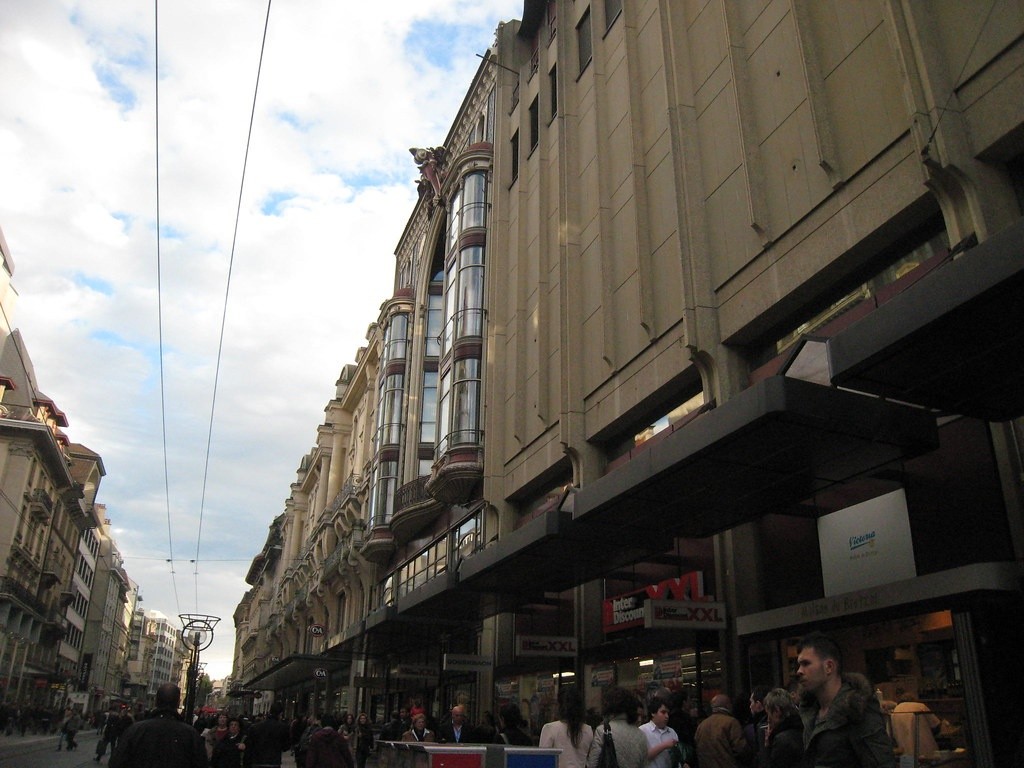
[57,677,73,723]
[178,613,221,727]
[87,685,98,724]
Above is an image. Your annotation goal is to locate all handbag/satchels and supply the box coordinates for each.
[598,721,619,768]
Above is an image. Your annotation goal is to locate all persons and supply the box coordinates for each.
[795,631,895,768]
[0,672,943,768]
